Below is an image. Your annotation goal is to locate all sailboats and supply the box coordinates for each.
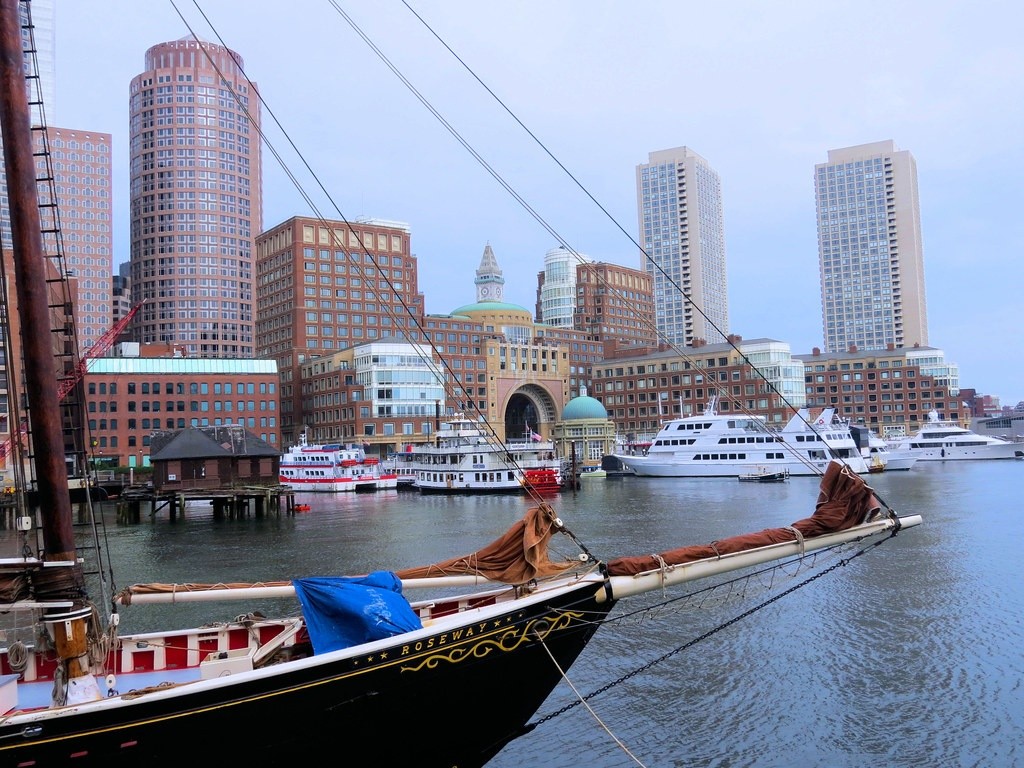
[0,1,930,767]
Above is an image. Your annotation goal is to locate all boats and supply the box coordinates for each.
[868,430,888,474]
[279,425,397,494]
[887,437,923,470]
[896,408,1024,462]
[378,443,415,489]
[737,465,789,483]
[412,413,565,494]
[612,394,870,478]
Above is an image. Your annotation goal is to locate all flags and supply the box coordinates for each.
[531,431,542,441]
[359,438,370,446]
